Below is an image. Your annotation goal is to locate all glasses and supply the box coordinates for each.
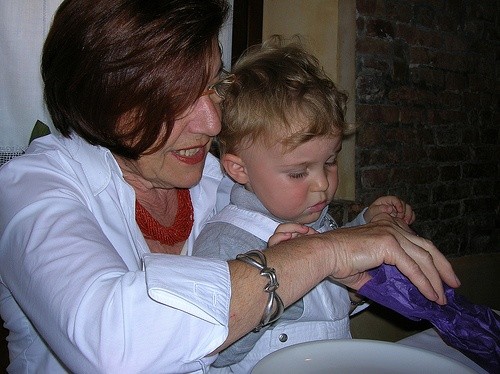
[196,68,236,98]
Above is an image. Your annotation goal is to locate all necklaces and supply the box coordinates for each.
[132,189,196,245]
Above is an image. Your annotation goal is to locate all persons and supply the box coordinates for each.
[190,35,417,374]
[0,0,460,374]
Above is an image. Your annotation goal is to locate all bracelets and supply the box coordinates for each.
[236,249,286,333]
